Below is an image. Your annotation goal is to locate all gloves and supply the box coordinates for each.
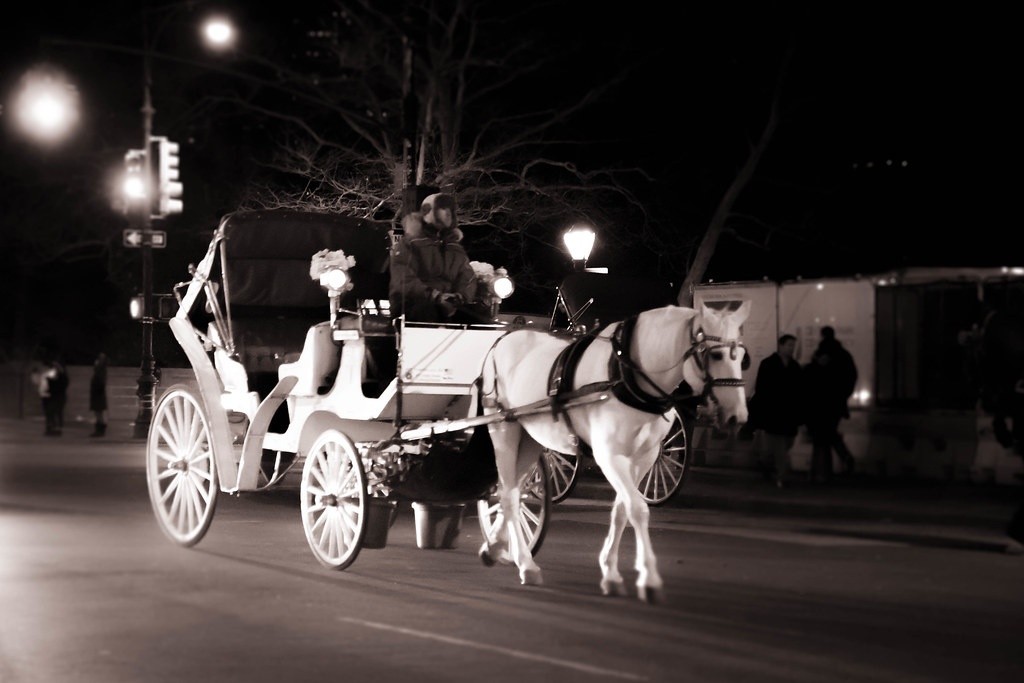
[438,292,464,311]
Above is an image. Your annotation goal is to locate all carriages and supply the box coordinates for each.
[146,206,752,604]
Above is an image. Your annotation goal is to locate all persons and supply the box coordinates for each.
[981,284,1024,542]
[39,361,68,436]
[91,353,108,436]
[803,327,857,485]
[390,193,477,322]
[756,335,802,480]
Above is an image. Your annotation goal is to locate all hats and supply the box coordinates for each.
[420,193,457,233]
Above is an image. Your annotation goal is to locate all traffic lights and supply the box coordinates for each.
[131,293,146,323]
[154,292,180,321]
[150,136,185,214]
[122,150,148,211]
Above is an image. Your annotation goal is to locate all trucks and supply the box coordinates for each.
[687,266,1024,485]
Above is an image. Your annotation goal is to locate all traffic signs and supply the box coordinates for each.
[122,229,167,248]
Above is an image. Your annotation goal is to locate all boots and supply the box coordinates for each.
[91,422,106,436]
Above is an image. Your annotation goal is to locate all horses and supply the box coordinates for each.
[476,291,753,604]
